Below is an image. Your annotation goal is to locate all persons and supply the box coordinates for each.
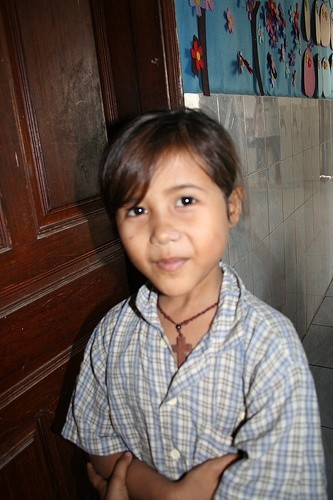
[61,109,327,500]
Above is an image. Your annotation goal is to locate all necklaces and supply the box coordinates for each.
[157,294,219,369]
